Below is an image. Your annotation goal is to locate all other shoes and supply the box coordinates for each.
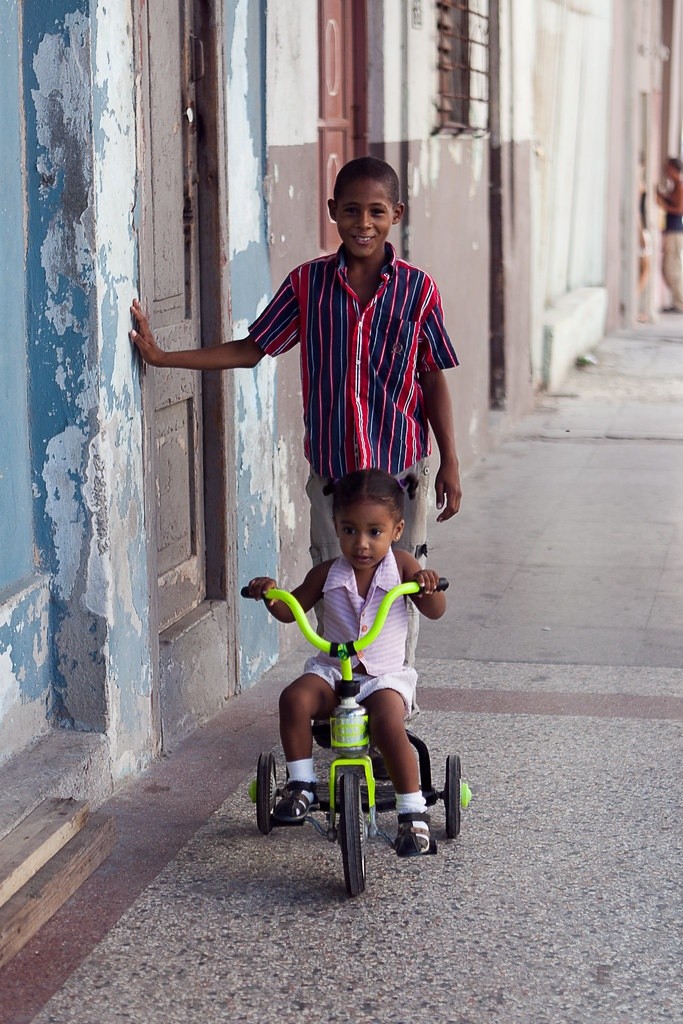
[662,305,683,313]
[371,755,392,781]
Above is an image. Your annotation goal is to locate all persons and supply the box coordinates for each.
[657,158,683,313]
[637,151,653,325]
[247,469,448,857]
[128,156,464,782]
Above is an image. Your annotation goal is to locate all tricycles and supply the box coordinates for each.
[244,576,474,897]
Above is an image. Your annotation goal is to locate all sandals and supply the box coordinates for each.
[272,780,321,822]
[396,811,432,856]
